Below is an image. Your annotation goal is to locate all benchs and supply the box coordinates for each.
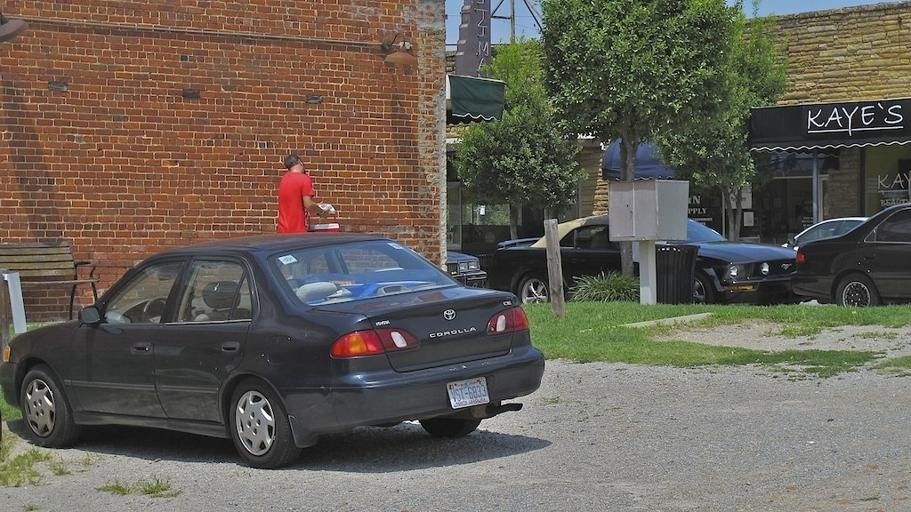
[0,240,99,327]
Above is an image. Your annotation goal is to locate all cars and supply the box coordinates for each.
[1,232,545,468]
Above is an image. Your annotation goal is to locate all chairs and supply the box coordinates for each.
[576,230,611,247]
[194,266,403,323]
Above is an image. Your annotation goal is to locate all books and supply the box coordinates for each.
[317,203,336,216]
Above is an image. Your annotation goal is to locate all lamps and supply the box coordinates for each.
[381,32,418,65]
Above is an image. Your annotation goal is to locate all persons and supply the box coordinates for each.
[275,154,330,275]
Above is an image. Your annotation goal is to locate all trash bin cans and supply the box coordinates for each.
[655,244,701,305]
